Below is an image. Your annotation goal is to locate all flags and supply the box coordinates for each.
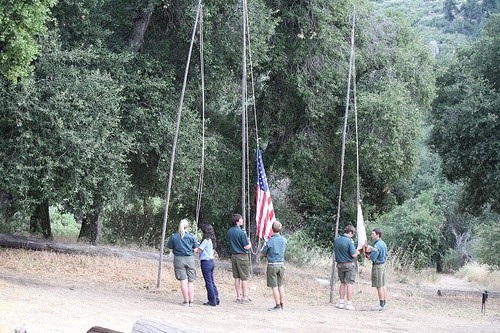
[357,202,371,258]
[255,144,277,239]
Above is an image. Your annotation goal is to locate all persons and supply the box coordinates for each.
[363,228,388,311]
[226,213,252,304]
[198,224,220,306]
[167,219,199,306]
[260,221,287,311]
[334,225,360,309]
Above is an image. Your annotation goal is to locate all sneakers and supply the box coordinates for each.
[237,299,241,302]
[268,308,281,313]
[346,304,354,309]
[372,306,385,311]
[336,303,344,308]
[242,298,254,304]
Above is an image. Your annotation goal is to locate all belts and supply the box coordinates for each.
[268,262,283,265]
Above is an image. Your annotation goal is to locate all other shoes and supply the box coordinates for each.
[179,301,189,306]
[216,302,219,305]
[204,303,216,307]
[189,300,193,306]
